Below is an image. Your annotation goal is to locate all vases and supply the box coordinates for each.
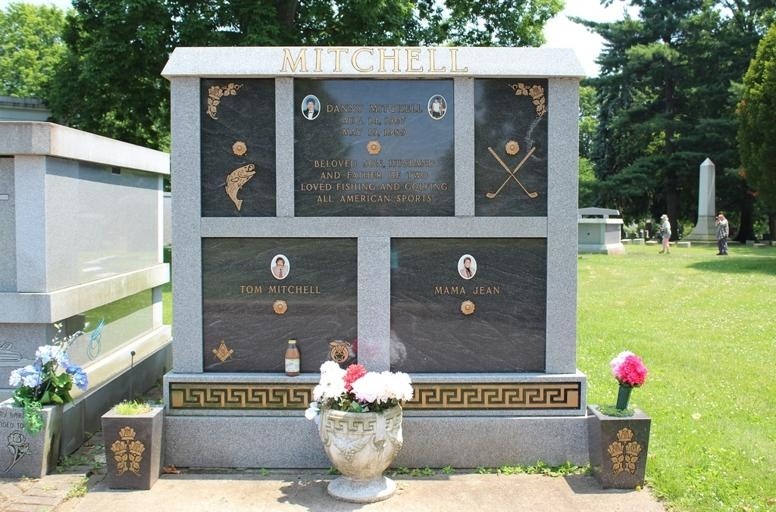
[584,403,653,492]
[318,405,404,502]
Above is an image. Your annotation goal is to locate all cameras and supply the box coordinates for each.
[715,217,718,221]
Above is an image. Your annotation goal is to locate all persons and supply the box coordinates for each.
[714,213,729,256]
[430,97,444,118]
[658,213,671,253]
[460,256,475,279]
[302,98,318,119]
[272,258,288,279]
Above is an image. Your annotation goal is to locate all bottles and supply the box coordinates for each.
[284,339,301,376]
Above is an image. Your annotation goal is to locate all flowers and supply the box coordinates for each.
[304,360,413,423]
[8,318,107,438]
[601,347,648,414]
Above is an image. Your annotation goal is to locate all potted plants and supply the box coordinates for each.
[101,400,166,491]
